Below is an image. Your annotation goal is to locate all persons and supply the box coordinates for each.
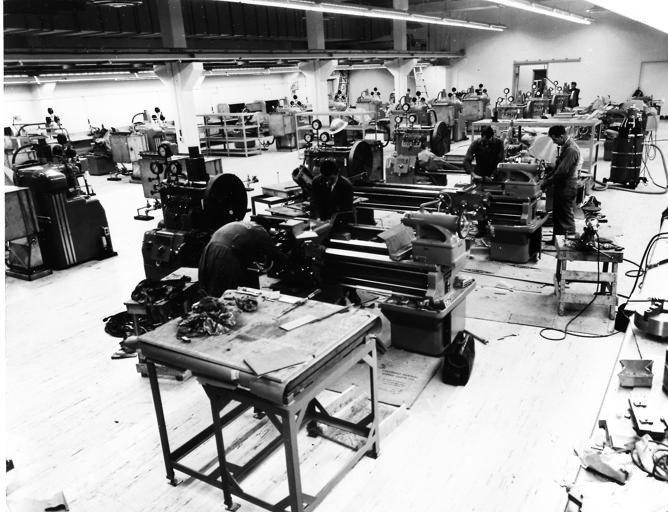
[537,126,585,246]
[567,82,580,107]
[462,125,503,185]
[195,216,276,297]
[309,159,353,220]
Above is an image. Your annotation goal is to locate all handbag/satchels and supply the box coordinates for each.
[442,329,475,387]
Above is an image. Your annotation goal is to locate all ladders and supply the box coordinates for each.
[413,67,430,99]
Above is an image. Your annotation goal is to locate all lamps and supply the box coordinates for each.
[492,1,594,26]
[227,0,509,33]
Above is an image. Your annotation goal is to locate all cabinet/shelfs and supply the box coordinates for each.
[473,117,602,189]
[197,113,262,158]
[295,110,377,153]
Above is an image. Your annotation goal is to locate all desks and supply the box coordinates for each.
[137,287,382,512]
[554,233,623,321]
